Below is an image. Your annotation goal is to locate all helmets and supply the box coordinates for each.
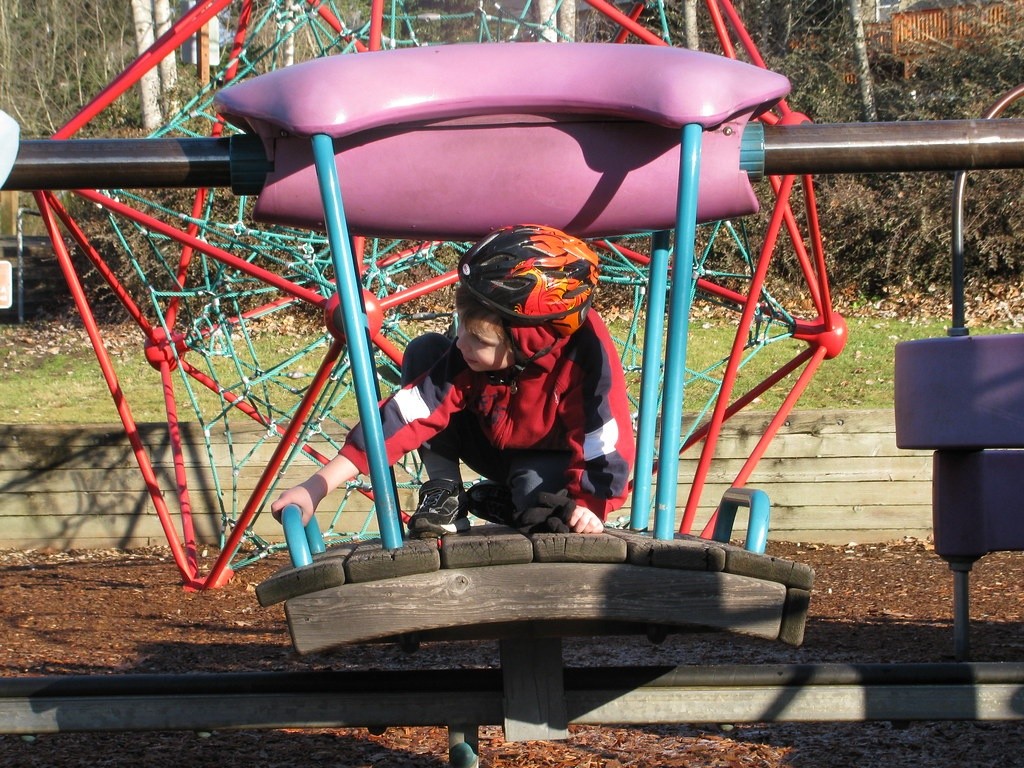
[457,224,600,339]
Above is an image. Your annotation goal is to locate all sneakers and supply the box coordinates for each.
[467,480,514,526]
[408,480,471,538]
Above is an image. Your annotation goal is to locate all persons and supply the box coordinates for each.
[271,225,636,535]
